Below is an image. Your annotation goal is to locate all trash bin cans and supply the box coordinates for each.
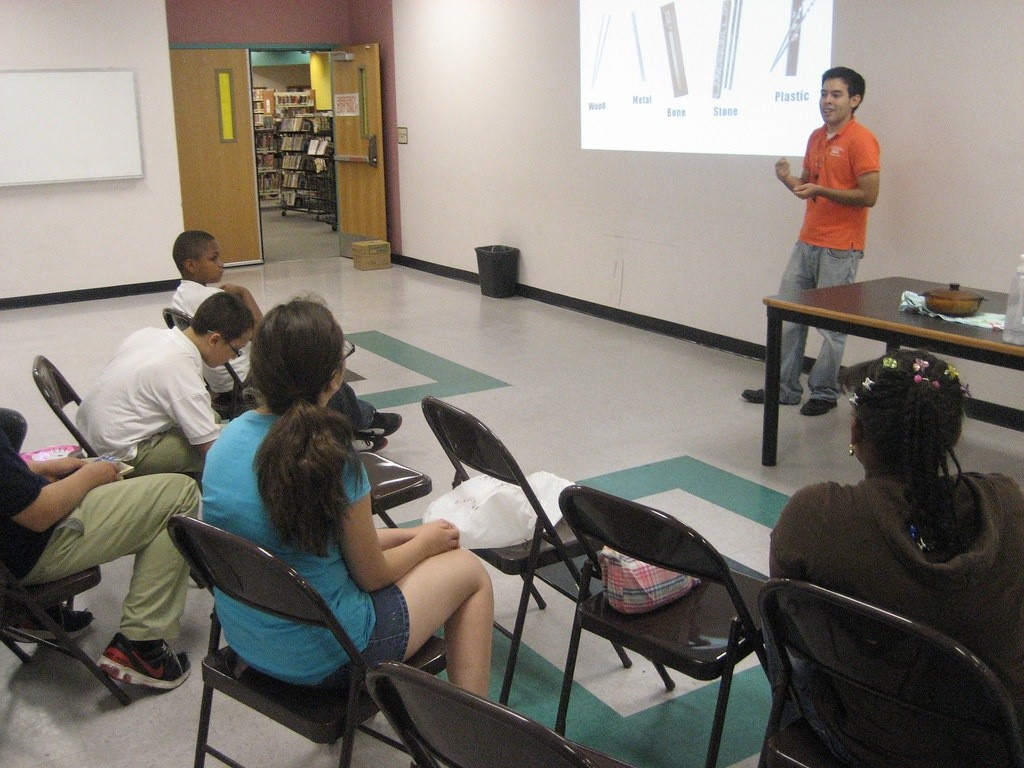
[474,245,519,299]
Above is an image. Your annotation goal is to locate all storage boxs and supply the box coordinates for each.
[352,240,391,271]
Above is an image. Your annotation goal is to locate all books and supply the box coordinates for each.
[253,88,331,207]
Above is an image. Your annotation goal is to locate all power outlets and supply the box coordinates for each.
[397,128,408,144]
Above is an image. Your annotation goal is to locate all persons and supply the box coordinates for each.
[0,424,203,690]
[200,289,494,699]
[77,292,255,490]
[741,67,880,416]
[769,349,1024,768]
[172,231,402,453]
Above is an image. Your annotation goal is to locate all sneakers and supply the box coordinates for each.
[801,398,838,416]
[353,407,402,436]
[2,600,94,642]
[349,429,388,453]
[742,388,800,405]
[96,630,191,689]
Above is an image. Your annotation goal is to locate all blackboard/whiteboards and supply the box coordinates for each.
[1,68,146,187]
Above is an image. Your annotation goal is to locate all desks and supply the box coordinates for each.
[761,277,1024,467]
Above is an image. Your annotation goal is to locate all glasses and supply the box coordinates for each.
[206,330,244,360]
[326,336,356,393]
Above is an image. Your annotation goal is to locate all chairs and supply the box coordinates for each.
[0,309,1024,768]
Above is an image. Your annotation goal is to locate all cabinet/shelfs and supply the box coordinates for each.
[251,89,338,230]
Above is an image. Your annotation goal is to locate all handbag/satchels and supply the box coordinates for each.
[596,537,700,613]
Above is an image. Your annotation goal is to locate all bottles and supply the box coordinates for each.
[1003,254,1024,346]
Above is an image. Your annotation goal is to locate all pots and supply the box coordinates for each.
[918,282,988,318]
